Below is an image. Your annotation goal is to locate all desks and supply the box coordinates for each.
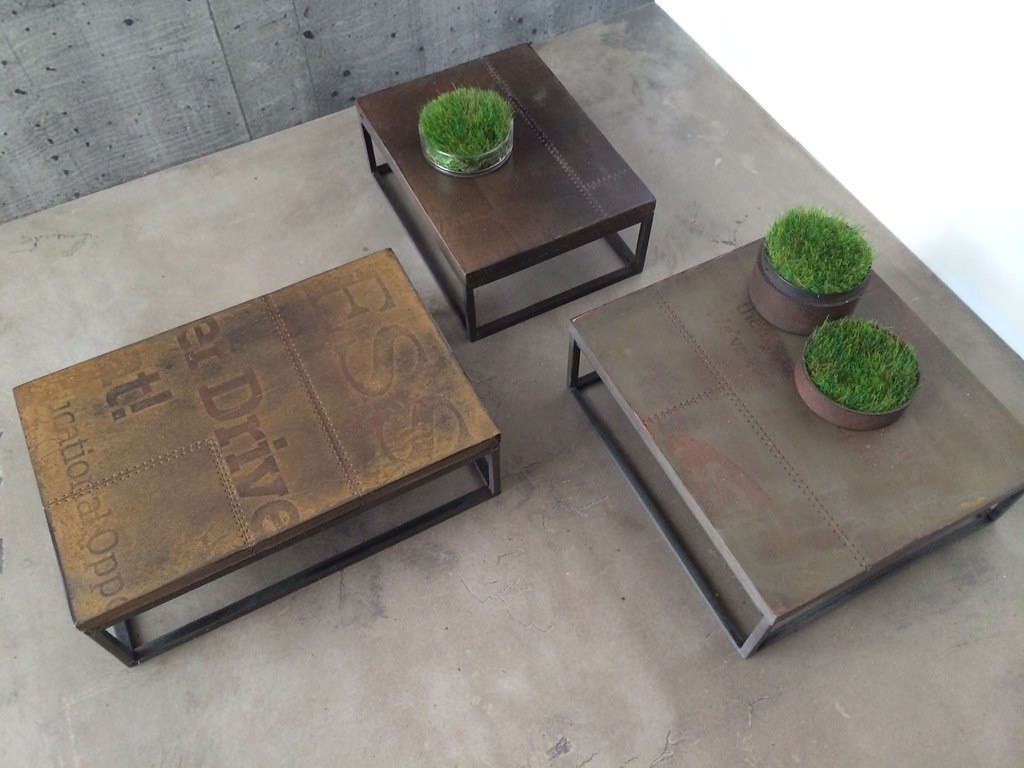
[355,44,657,343]
[567,236,1024,659]
[12,248,503,667]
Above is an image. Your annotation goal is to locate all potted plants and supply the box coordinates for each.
[794,313,922,431]
[417,81,517,177]
[747,203,875,337]
